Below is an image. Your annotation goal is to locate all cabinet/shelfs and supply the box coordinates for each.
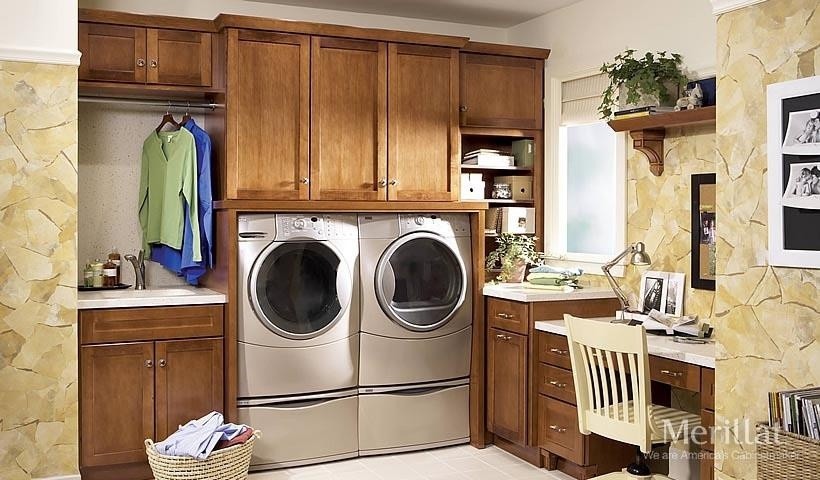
[204,12,309,201]
[308,24,458,204]
[459,36,552,272]
[476,281,623,447]
[79,302,227,478]
[78,11,219,95]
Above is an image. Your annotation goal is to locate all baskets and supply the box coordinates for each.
[144,426,256,480]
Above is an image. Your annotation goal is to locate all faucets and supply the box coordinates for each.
[124,249,146,291]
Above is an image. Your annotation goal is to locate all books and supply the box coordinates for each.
[461,154,515,167]
[641,308,699,338]
[464,149,511,157]
[767,384,820,441]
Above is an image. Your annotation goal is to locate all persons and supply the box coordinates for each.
[791,112,820,196]
[644,280,661,311]
[700,213,716,244]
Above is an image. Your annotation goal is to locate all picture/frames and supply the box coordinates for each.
[661,270,687,317]
[691,172,715,292]
[766,76,820,272]
[637,268,670,315]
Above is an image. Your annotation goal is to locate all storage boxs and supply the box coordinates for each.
[510,139,534,169]
[497,206,538,236]
[493,175,532,202]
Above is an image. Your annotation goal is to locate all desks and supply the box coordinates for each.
[533,307,714,480]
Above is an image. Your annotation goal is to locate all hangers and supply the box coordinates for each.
[150,100,179,135]
[175,100,196,131]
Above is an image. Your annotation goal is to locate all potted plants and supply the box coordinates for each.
[593,47,707,122]
[484,228,547,284]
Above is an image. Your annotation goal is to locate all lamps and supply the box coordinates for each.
[602,242,654,327]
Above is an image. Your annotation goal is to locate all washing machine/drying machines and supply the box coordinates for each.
[357,213,473,457]
[237,212,359,472]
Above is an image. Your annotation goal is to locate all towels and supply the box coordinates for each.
[84,245,121,289]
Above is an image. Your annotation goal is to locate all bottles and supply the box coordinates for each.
[82,263,93,287]
[702,220,715,244]
[91,259,103,287]
[102,259,119,287]
[492,183,512,199]
[108,246,121,273]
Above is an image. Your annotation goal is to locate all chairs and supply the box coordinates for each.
[563,310,702,480]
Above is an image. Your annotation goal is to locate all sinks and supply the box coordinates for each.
[102,289,196,300]
